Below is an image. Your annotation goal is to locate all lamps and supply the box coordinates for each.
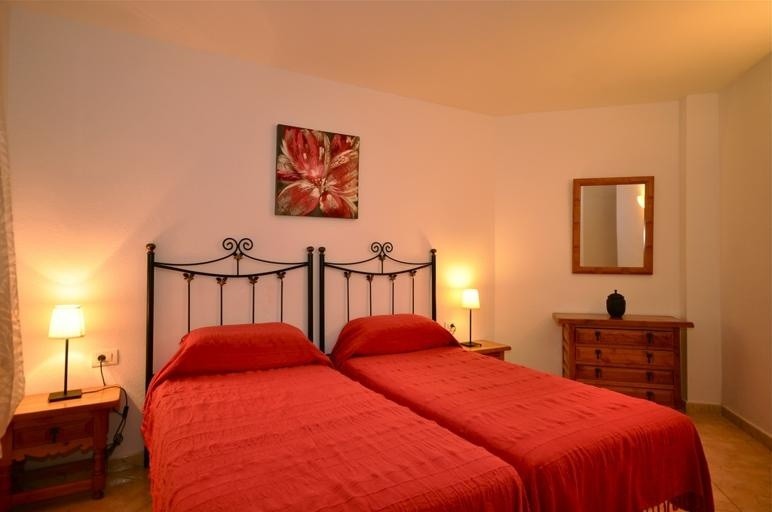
[48,305,85,402]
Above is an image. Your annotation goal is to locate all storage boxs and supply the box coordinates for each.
[462,289,482,348]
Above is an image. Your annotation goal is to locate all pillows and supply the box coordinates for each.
[331,314,460,357]
[166,323,328,374]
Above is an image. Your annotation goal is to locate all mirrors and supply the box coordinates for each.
[572,176,654,275]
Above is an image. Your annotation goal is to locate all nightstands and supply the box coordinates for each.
[463,341,512,361]
[0,385,121,512]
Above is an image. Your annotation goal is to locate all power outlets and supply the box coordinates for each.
[91,348,119,368]
[445,321,455,331]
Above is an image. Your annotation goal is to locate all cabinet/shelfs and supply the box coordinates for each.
[553,312,694,411]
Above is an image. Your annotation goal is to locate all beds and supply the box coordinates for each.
[140,240,531,512]
[318,242,715,512]
